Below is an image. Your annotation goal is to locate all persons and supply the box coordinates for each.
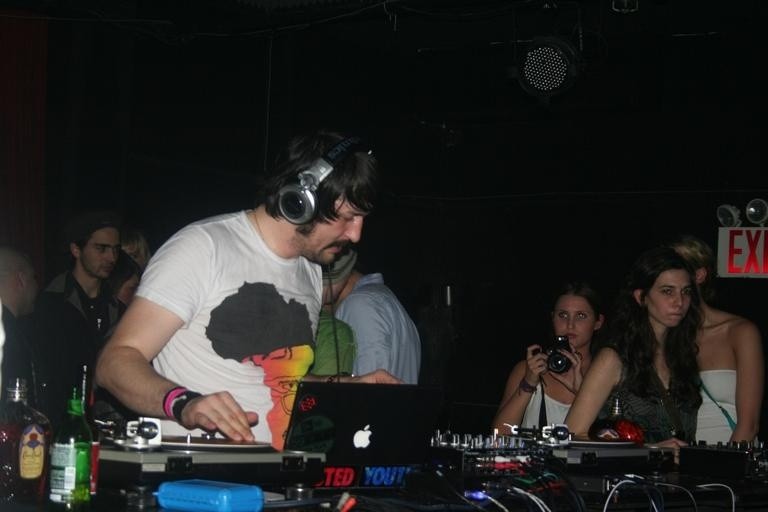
[657,237,765,448]
[103,248,143,329]
[322,262,423,385]
[560,249,707,465]
[308,242,361,380]
[493,283,609,445]
[115,226,151,270]
[93,128,386,455]
[0,242,53,416]
[41,220,127,438]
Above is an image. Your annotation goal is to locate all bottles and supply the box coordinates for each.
[1,365,99,511]
[607,397,625,429]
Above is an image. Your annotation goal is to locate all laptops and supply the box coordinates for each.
[265,380,447,501]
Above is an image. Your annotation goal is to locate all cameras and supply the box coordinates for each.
[534,335,577,373]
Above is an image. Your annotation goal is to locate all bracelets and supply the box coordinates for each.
[328,370,355,383]
[162,386,187,420]
[170,390,203,429]
[517,376,538,395]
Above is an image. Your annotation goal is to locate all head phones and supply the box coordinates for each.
[275,133,373,223]
